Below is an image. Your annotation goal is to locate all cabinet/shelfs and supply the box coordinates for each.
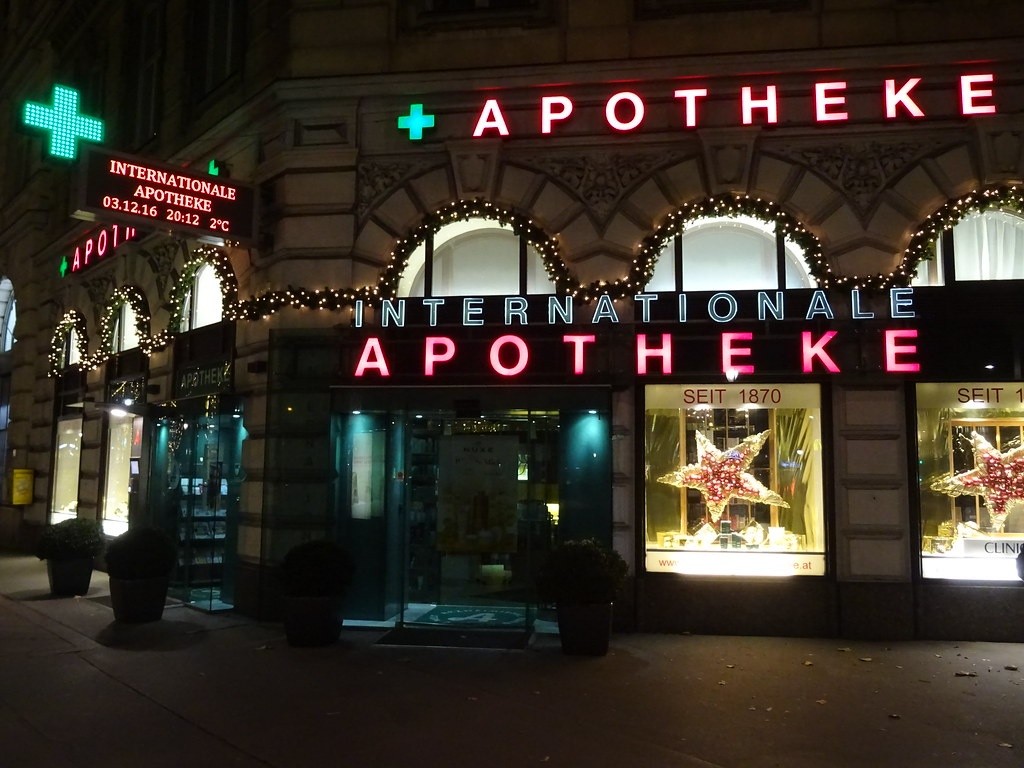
[175,493,229,587]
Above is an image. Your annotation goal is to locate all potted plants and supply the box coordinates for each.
[106,527,175,622]
[34,518,105,597]
